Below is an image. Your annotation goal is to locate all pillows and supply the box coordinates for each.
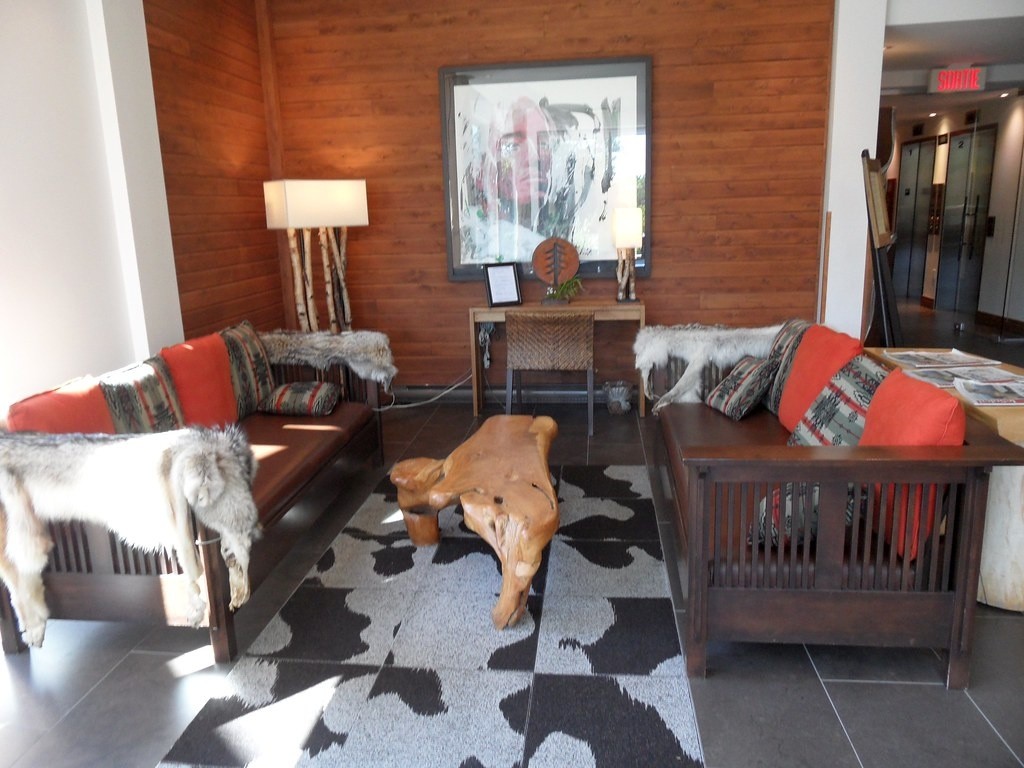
[748,481,866,550]
[863,365,967,562]
[763,321,813,412]
[99,356,184,436]
[4,374,115,435]
[252,381,340,415]
[217,320,275,419]
[160,332,238,431]
[704,355,777,421]
[786,354,890,453]
[776,323,863,434]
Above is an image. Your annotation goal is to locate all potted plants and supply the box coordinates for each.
[540,275,583,305]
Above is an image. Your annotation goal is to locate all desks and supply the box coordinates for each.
[468,299,647,420]
[864,347,1024,441]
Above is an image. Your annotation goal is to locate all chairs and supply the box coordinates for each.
[502,308,597,438]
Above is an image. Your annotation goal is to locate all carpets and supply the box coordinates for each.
[160,463,707,768]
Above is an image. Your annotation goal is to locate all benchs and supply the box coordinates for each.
[0,321,388,667]
[631,325,1024,690]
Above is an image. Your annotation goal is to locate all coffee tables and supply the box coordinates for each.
[396,413,560,631]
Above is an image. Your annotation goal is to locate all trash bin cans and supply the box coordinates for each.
[604,379,634,416]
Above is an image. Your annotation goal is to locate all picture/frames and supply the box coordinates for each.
[482,262,522,308]
[438,54,652,282]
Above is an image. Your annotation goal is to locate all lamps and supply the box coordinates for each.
[263,177,370,328]
